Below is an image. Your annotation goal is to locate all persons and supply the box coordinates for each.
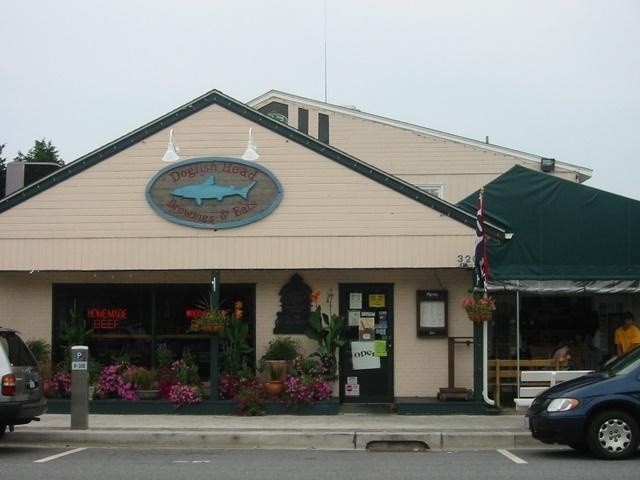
[613,311,640,359]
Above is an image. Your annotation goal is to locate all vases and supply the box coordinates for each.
[468,311,493,321]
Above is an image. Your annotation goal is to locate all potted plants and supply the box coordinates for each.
[263,339,296,374]
[200,382,223,399]
[190,290,225,333]
[134,370,159,400]
[265,368,283,401]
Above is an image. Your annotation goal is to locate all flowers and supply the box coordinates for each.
[219,300,255,376]
[305,288,346,371]
[162,377,199,402]
[287,374,332,401]
[97,363,138,399]
[463,295,497,312]
[223,378,262,402]
[165,357,189,371]
[46,369,73,395]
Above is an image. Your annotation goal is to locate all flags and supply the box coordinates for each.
[471,194,494,290]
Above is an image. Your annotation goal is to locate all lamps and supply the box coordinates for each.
[541,158,556,172]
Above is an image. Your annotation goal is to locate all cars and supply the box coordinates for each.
[0,327,48,438]
[526,344,640,459]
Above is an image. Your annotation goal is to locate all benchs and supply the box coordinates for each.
[486,359,562,409]
[514,370,598,413]
